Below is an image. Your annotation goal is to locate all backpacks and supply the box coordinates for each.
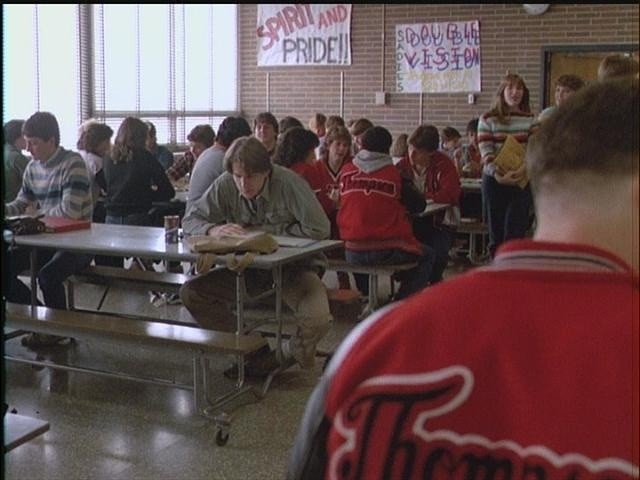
[185,232,279,275]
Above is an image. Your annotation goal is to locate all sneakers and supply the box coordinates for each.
[224,343,299,379]
[21,330,72,348]
[148,290,182,308]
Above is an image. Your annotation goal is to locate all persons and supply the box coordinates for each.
[539,74,587,122]
[4,119,30,202]
[598,53,640,83]
[177,136,333,383]
[284,73,640,480]
[478,74,538,261]
[77,117,182,222]
[166,111,280,216]
[278,113,480,305]
[2,113,95,346]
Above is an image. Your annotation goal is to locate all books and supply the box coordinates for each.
[39,217,91,234]
